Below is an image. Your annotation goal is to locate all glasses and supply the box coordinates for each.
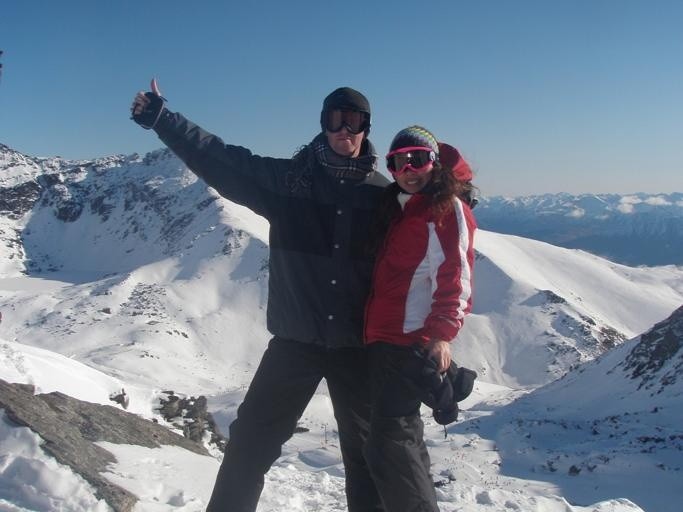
[321,108,369,134]
[386,147,435,176]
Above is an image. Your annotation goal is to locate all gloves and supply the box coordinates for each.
[404,342,476,425]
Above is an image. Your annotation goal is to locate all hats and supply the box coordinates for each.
[323,88,371,140]
[390,126,439,167]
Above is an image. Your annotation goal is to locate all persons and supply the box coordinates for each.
[128,78,480,511]
[361,125,476,511]
